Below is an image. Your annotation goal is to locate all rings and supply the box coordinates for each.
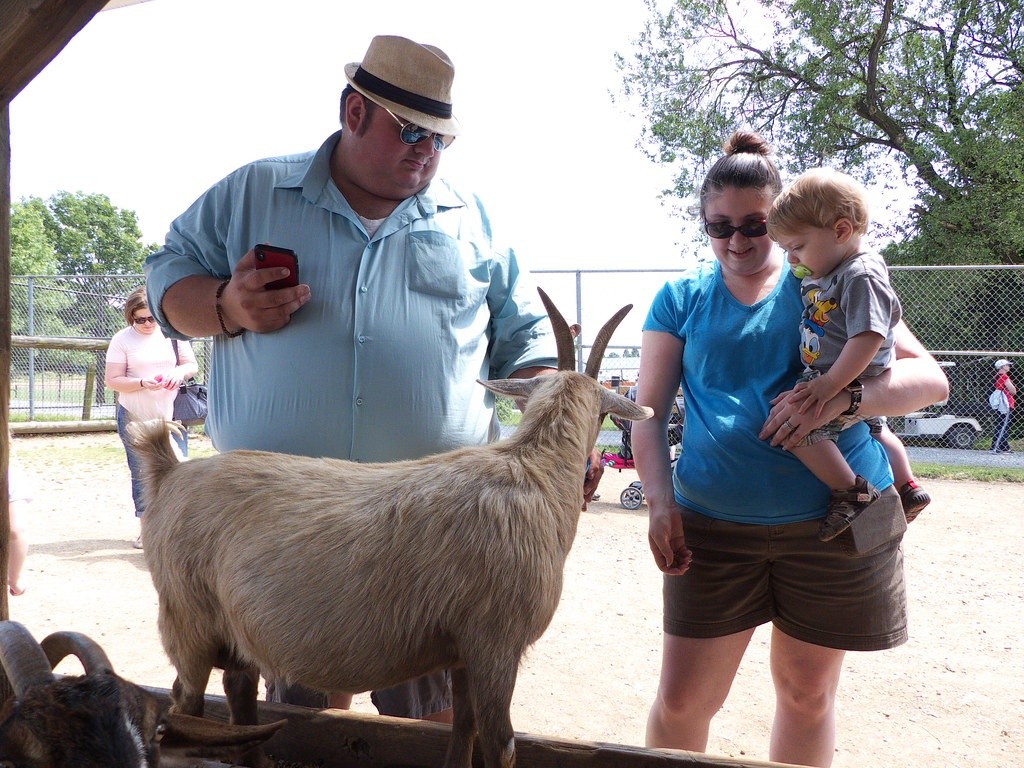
[784,420,797,430]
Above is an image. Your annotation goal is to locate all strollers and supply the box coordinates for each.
[608,384,685,510]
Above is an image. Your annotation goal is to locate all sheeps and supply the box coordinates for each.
[0,285,655,768]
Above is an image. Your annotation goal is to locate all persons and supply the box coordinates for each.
[145,34,561,726]
[988,360,1020,456]
[104,287,198,549]
[764,167,931,543]
[628,131,950,768]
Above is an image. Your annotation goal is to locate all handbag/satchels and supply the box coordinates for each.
[170,338,208,426]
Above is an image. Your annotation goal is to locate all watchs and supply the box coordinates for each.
[840,378,864,415]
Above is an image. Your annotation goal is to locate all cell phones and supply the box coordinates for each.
[254,244,299,291]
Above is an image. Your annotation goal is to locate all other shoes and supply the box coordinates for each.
[135,536,143,549]
[988,450,1002,454]
[1003,450,1014,454]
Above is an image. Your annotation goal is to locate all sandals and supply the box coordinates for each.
[818,475,881,541]
[899,479,931,524]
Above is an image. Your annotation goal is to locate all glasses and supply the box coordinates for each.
[384,108,456,152]
[130,315,155,324]
[703,217,767,239]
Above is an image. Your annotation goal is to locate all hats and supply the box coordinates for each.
[995,360,1015,368]
[345,35,461,135]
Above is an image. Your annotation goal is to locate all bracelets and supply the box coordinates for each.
[140,378,144,388]
[215,279,247,338]
